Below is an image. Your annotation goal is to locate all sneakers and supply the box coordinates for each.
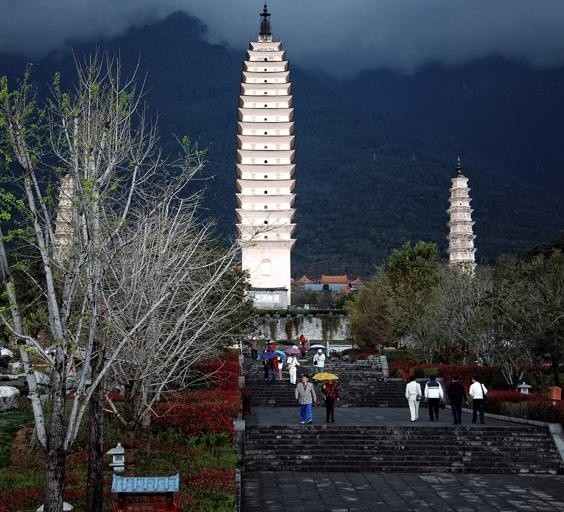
[301,421,305,424]
[307,420,312,424]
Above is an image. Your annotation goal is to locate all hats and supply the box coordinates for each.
[318,349,322,353]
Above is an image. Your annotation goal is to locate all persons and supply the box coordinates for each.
[469,376,488,424]
[250,332,340,424]
[404,375,422,422]
[448,376,468,424]
[424,375,444,421]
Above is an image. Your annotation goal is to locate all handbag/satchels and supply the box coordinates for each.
[416,395,422,401]
[441,402,445,408]
[313,361,318,365]
[484,394,488,401]
[286,365,291,370]
